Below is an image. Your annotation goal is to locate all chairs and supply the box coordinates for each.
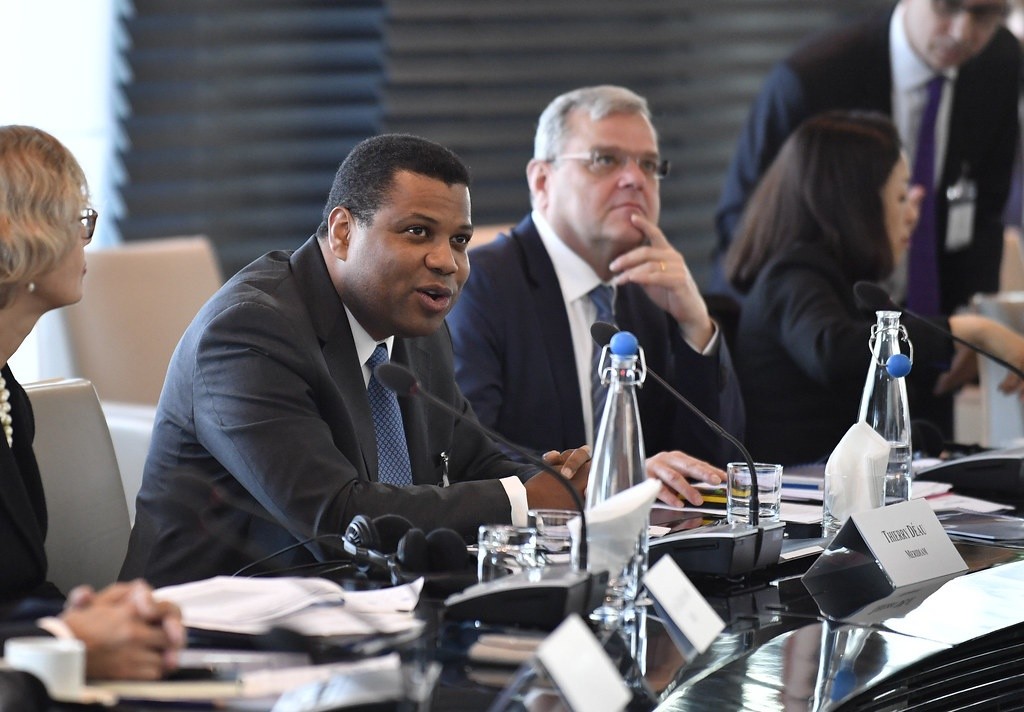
[15,376,131,598]
[56,236,224,407]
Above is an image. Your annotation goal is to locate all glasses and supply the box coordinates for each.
[76,208,99,239]
[929,0,1013,27]
[542,148,670,180]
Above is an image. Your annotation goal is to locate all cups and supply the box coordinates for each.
[7,638,86,695]
[528,508,580,568]
[975,295,1024,446]
[570,536,638,604]
[820,472,886,539]
[726,461,784,526]
[477,525,535,584]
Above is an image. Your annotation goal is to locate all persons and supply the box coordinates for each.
[721,110,1024,469]
[443,84,748,510]
[712,0,1024,447]
[0,126,185,712]
[116,133,591,590]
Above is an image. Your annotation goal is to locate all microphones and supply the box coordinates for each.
[589,321,787,580]
[850,281,1024,506]
[373,361,609,632]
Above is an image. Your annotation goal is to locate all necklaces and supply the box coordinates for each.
[0,372,13,448]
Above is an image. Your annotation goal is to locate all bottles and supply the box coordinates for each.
[856,309,914,507]
[586,354,649,613]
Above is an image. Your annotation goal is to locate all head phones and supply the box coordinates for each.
[342,515,413,568]
[387,529,472,599]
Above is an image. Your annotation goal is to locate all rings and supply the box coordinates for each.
[660,261,665,271]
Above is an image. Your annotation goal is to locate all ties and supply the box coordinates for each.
[365,344,413,489]
[586,284,621,455]
[905,75,948,317]
